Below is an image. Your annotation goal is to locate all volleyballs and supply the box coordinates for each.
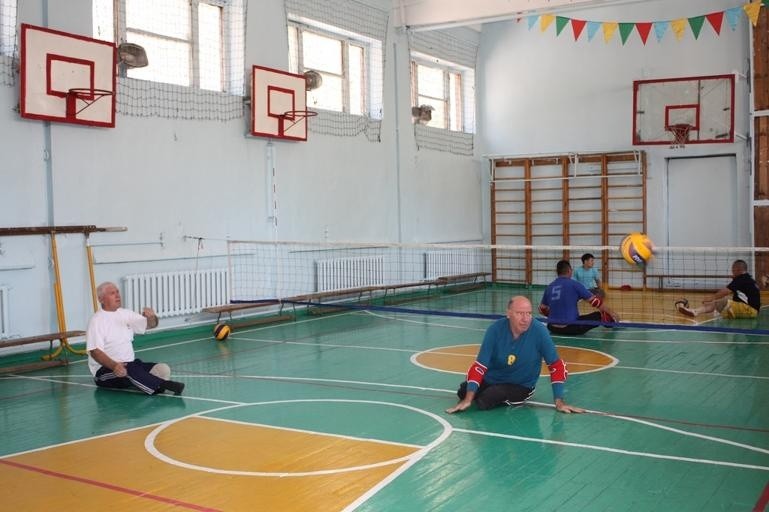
[214,323,230,340]
[621,233,650,264]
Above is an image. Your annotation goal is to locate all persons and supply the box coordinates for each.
[539,260,618,336]
[445,296,584,414]
[572,254,605,300]
[85,282,184,395]
[679,259,760,320]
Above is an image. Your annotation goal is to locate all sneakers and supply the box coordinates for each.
[166,382,184,395]
[679,307,696,317]
[153,388,164,395]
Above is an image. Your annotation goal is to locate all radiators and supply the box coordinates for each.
[315,256,385,292]
[426,251,490,280]
[121,269,231,318]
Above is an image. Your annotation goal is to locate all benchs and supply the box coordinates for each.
[201,271,493,332]
